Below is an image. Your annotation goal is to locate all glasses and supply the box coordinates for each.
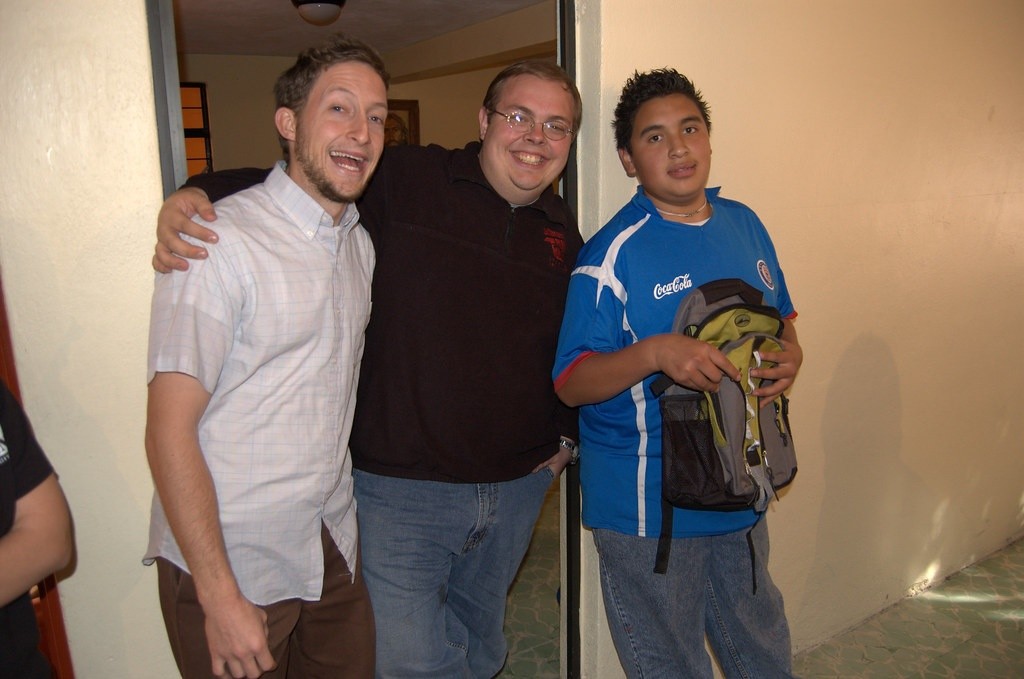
[486,107,576,141]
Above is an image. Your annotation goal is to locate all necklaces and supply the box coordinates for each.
[656,196,707,217]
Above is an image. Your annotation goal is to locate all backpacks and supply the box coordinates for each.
[649,278,798,596]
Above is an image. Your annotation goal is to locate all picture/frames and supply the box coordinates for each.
[385,100,420,148]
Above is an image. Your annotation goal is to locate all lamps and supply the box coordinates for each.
[290,0,346,26]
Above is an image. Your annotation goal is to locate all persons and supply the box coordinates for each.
[151,53,593,679]
[0,382,76,679]
[550,66,802,679]
[143,32,385,678]
[558,438,579,465]
